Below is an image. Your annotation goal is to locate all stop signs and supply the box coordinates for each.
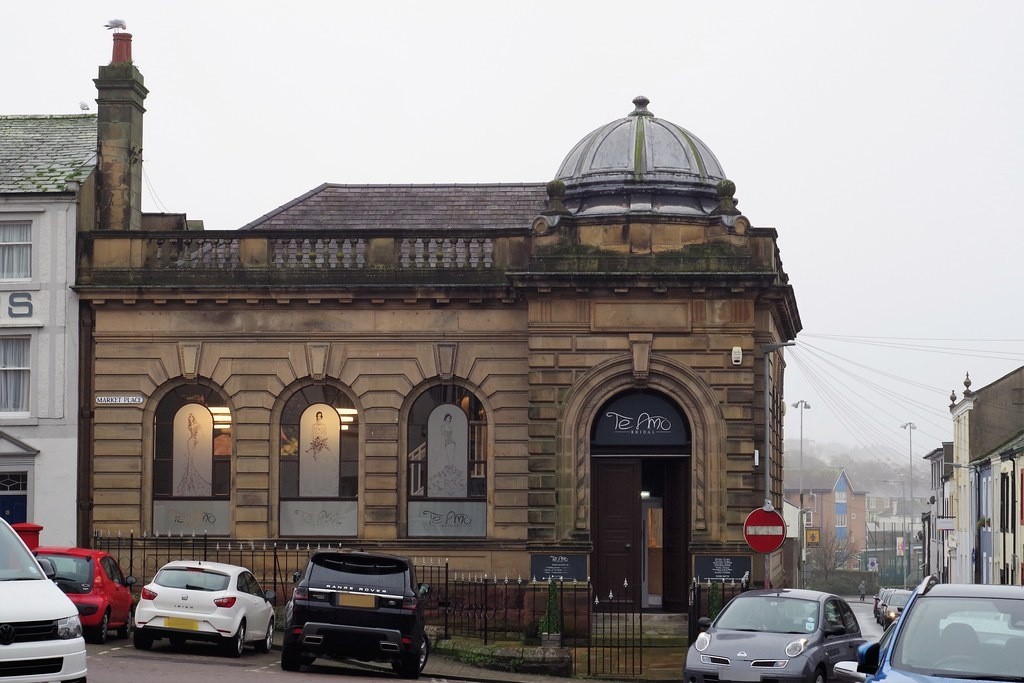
[743,508,788,555]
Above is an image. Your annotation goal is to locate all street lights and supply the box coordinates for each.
[794,398,810,586]
[901,421,915,584]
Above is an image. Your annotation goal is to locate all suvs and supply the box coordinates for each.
[281,549,427,680]
[832,575,1024,683]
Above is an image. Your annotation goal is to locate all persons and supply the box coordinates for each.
[858,581,865,601]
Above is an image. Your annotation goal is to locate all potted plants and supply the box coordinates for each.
[539,579,561,647]
[986,518,990,527]
[977,515,986,528]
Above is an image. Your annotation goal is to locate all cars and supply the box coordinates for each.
[686,587,866,683]
[874,588,917,629]
[0,517,133,683]
[134,561,274,659]
